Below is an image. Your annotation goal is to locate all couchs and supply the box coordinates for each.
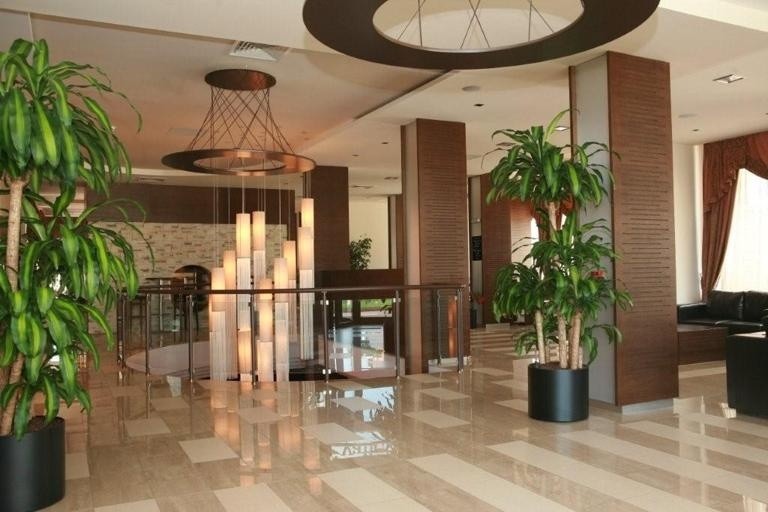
[677,289,768,419]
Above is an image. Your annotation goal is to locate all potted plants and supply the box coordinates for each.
[481,109,634,423]
[0,36,156,512]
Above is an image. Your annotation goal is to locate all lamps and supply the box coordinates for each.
[208,381,324,497]
[161,67,317,382]
